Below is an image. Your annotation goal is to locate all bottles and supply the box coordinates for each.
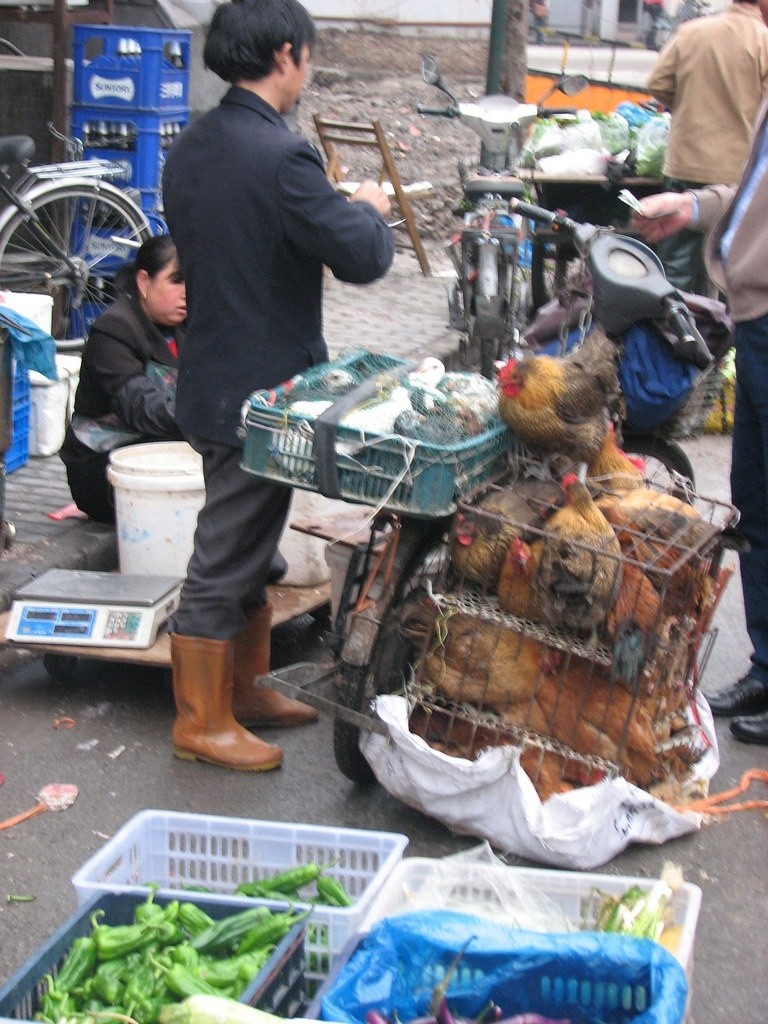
[68,26,186,302]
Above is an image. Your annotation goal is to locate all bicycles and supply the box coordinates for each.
[0,134,154,353]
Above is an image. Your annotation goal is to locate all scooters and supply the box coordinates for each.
[298,200,715,791]
[414,54,588,378]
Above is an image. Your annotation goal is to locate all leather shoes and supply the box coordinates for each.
[730,715,768,745]
[703,675,768,716]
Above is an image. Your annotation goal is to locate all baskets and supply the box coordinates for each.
[0,810,702,1024]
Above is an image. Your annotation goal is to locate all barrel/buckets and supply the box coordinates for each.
[267,488,349,588]
[105,441,209,582]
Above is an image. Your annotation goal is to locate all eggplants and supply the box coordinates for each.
[367,935,581,1024]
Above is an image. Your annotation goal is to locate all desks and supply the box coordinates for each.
[514,161,663,215]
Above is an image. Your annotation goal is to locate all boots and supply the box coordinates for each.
[232,603,319,728]
[170,632,284,773]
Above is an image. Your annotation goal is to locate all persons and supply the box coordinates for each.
[59,233,186,523]
[645,0,768,294]
[630,98,768,746]
[167,0,395,772]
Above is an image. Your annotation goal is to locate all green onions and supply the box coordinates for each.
[602,863,682,947]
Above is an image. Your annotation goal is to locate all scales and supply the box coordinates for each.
[7,569,186,649]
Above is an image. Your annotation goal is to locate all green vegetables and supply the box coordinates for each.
[517,113,670,179]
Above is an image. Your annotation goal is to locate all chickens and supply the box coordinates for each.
[533,473,622,650]
[371,426,728,810]
[495,333,620,487]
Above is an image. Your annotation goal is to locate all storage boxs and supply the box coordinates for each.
[2,365,31,474]
[241,352,511,518]
[26,352,79,459]
[302,933,689,1024]
[66,805,408,992]
[60,22,195,341]
[0,891,308,1024]
[0,289,55,343]
[360,859,702,985]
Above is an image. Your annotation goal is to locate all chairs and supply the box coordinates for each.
[309,111,437,279]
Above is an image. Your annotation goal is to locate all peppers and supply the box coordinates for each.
[29,859,355,1024]
